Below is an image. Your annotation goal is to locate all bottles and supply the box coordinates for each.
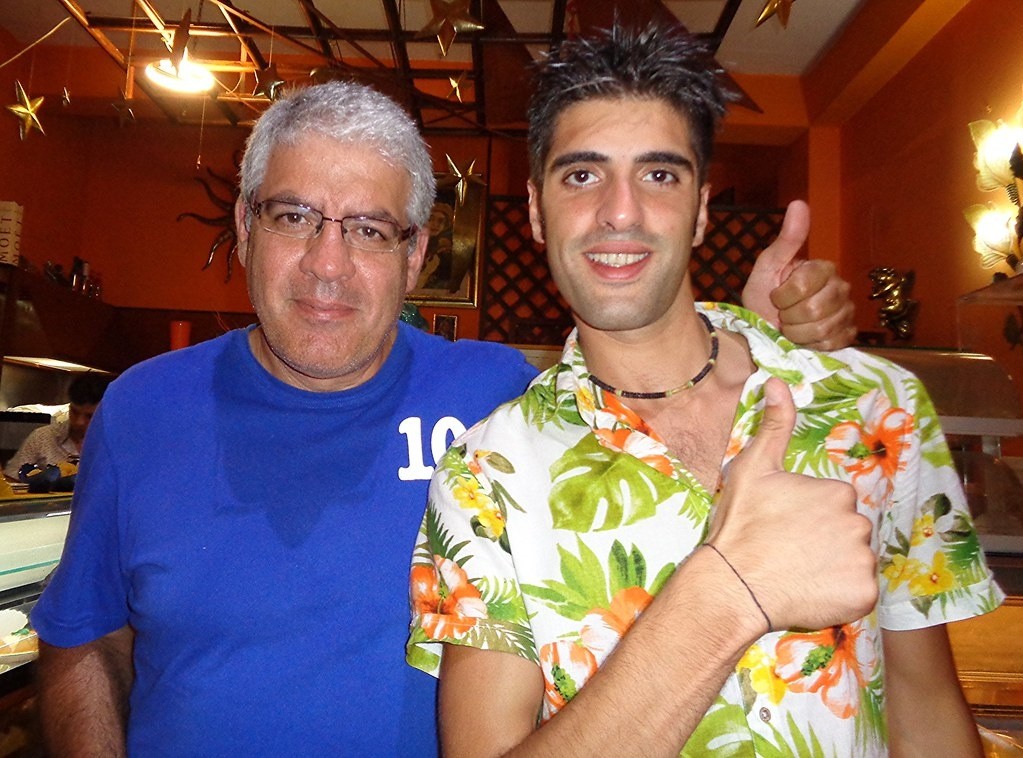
[69,255,103,299]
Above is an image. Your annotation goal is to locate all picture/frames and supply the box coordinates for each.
[432,313,458,343]
[402,171,484,309]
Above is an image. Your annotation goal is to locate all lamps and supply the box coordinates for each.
[955,101,1023,307]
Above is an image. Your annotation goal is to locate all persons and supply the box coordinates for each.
[5,370,115,487]
[29,78,863,756]
[402,67,1006,756]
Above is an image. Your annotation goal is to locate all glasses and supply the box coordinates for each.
[248,190,418,253]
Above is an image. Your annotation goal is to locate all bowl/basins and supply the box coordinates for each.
[19,461,61,496]
[50,474,77,492]
[10,482,29,494]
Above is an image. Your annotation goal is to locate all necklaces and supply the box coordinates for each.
[564,310,725,399]
